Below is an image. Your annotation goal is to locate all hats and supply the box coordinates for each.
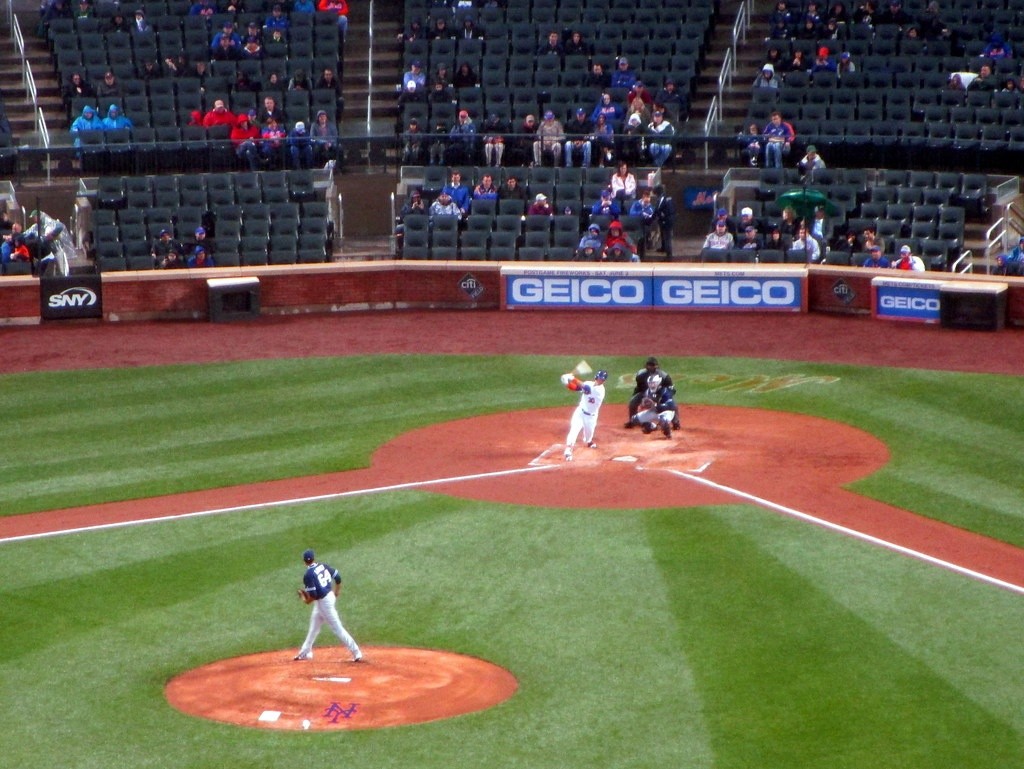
[544,110,554,120]
[526,115,535,122]
[105,72,113,79]
[160,229,169,234]
[459,111,468,117]
[295,122,304,133]
[215,100,223,108]
[195,227,205,234]
[302,549,314,561]
[576,107,585,115]
[820,47,828,55]
[536,193,547,201]
[716,208,754,231]
[29,210,40,220]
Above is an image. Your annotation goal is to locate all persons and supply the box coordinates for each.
[189,67,346,173]
[515,32,685,169]
[0,209,70,276]
[699,206,1024,277]
[394,161,676,262]
[752,0,1024,104]
[400,110,511,168]
[149,226,214,269]
[625,357,680,439]
[561,370,608,461]
[39,0,348,94]
[398,0,485,105]
[294,550,362,662]
[745,111,826,184]
[63,72,133,158]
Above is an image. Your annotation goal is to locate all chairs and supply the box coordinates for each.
[0,0,1024,272]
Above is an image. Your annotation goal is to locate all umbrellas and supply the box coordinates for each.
[774,188,839,219]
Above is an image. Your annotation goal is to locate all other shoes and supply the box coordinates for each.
[293,655,313,660]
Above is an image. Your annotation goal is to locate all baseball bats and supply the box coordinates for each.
[563,360,592,380]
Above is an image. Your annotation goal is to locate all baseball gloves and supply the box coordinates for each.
[298,589,311,604]
[643,398,655,408]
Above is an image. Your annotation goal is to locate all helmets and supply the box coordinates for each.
[595,370,608,380]
[645,357,658,370]
[647,374,662,393]
[841,52,851,60]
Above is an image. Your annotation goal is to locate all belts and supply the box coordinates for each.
[582,409,594,416]
